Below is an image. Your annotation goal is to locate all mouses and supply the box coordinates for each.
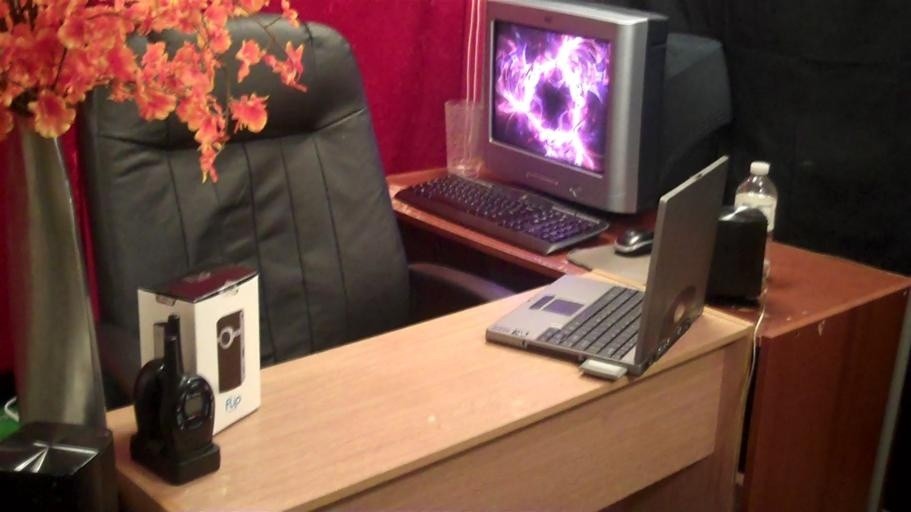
[612,219,654,256]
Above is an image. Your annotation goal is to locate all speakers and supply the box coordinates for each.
[704,206,768,308]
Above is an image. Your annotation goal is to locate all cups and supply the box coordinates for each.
[445,99,486,181]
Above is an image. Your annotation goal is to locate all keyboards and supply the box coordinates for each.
[393,170,612,259]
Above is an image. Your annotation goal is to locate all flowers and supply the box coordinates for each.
[0,0,317,186]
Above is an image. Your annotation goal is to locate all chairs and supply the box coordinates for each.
[71,12,520,416]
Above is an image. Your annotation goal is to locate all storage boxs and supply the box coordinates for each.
[134,263,263,442]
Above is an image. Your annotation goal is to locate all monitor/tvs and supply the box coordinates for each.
[484,0,734,216]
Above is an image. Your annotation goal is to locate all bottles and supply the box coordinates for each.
[731,160,779,285]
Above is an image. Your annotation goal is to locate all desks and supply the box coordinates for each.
[372,160,905,509]
[104,262,754,511]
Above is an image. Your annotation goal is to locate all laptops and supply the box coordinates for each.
[485,153,730,382]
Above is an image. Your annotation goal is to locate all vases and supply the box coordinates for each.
[12,92,120,511]
